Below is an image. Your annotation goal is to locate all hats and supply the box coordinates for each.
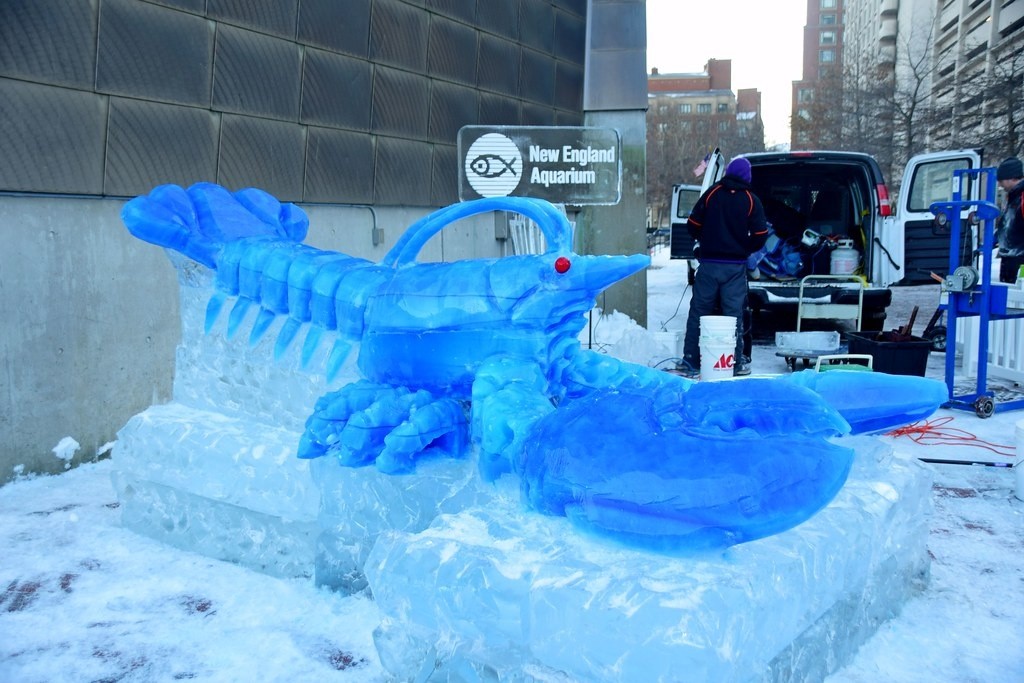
[997,157,1023,180]
[725,158,751,184]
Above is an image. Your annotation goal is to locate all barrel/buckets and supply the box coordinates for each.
[697,315,739,382]
[844,330,934,377]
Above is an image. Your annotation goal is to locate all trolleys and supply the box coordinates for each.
[775,275,864,372]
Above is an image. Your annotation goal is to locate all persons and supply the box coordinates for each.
[677,158,768,375]
[972,158,1024,285]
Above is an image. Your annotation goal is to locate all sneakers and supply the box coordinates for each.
[733,363,751,375]
[662,365,700,381]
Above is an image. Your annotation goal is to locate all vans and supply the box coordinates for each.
[670,146,985,332]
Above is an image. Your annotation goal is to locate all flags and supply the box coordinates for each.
[693,153,712,177]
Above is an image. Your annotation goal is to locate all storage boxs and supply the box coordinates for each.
[844,331,935,377]
